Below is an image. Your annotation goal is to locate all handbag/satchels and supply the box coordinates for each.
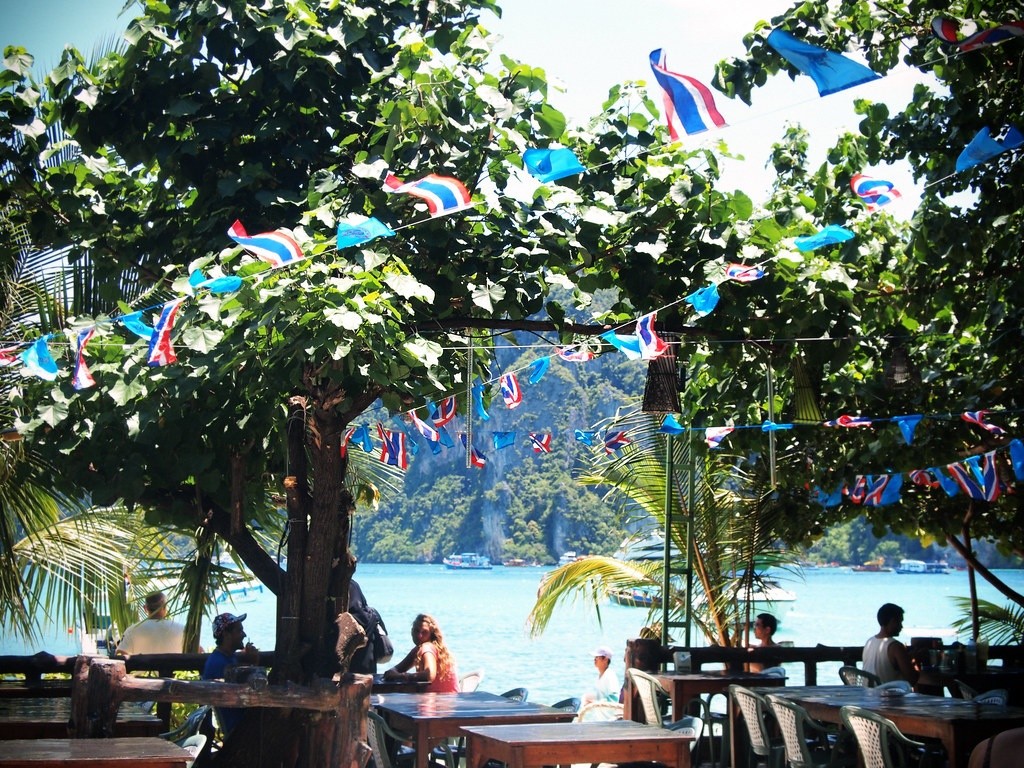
[374,629,395,663]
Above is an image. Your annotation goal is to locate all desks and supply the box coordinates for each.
[372,674,578,768]
[653,672,789,768]
[0,697,194,768]
[728,684,1024,768]
[459,721,695,768]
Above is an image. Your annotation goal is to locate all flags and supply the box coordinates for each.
[816,439,1024,507]
[0,311,154,391]
[336,217,396,250]
[146,299,183,367]
[227,219,305,269]
[522,148,587,184]
[188,269,242,293]
[381,174,473,218]
[930,15,1024,58]
[765,27,883,97]
[340,124,1024,471]
[649,48,730,142]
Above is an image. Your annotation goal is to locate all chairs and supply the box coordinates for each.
[367,668,1009,768]
[160,705,212,768]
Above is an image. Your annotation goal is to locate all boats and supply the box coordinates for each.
[442,551,494,570]
[502,559,528,567]
[558,552,578,567]
[75,603,119,656]
[605,587,677,609]
[895,559,951,575]
[798,558,841,568]
[851,556,893,572]
[699,579,798,604]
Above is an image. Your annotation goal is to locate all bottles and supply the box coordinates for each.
[966,638,977,670]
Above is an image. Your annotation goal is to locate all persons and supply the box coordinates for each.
[384,614,461,693]
[861,603,920,688]
[203,613,258,734]
[115,591,205,656]
[748,613,783,673]
[348,579,388,674]
[619,638,668,725]
[581,646,620,707]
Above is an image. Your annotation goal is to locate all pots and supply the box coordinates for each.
[929,644,963,668]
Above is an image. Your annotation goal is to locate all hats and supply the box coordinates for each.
[640,621,674,642]
[590,647,613,660]
[213,613,247,638]
